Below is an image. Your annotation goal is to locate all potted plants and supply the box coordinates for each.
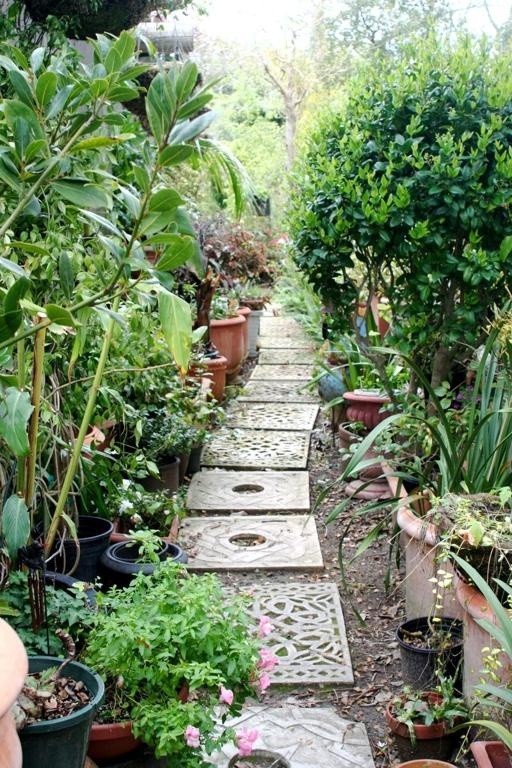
[284,18,512,768]
[0,33,266,768]
